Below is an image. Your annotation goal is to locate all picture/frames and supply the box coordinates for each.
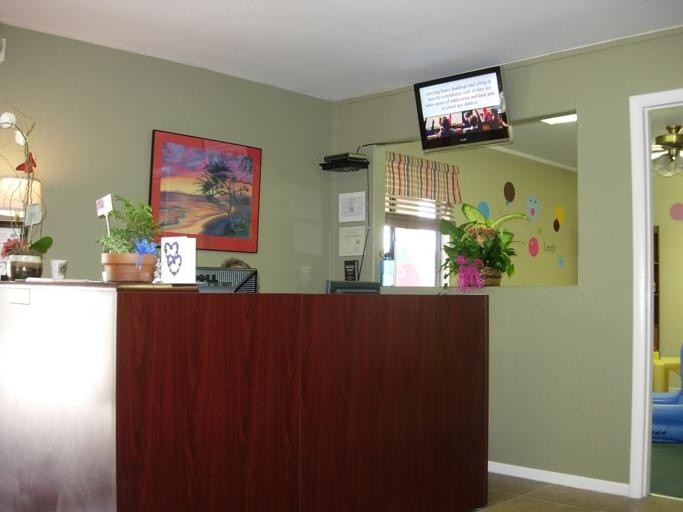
[148,129,262,253]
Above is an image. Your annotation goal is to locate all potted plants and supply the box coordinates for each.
[95,194,164,283]
[439,203,529,286]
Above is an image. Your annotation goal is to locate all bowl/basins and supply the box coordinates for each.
[10,261,42,281]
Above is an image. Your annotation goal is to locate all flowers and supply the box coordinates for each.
[1,236,53,256]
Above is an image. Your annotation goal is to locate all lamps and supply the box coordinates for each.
[0,175,42,221]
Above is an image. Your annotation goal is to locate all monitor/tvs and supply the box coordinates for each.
[413,65,514,153]
[173,267,257,293]
[326,279,380,293]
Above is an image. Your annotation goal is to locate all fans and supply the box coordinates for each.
[651,125,683,177]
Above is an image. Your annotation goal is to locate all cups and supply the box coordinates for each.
[51,260,68,280]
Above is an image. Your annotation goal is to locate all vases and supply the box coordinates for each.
[7,255,43,280]
